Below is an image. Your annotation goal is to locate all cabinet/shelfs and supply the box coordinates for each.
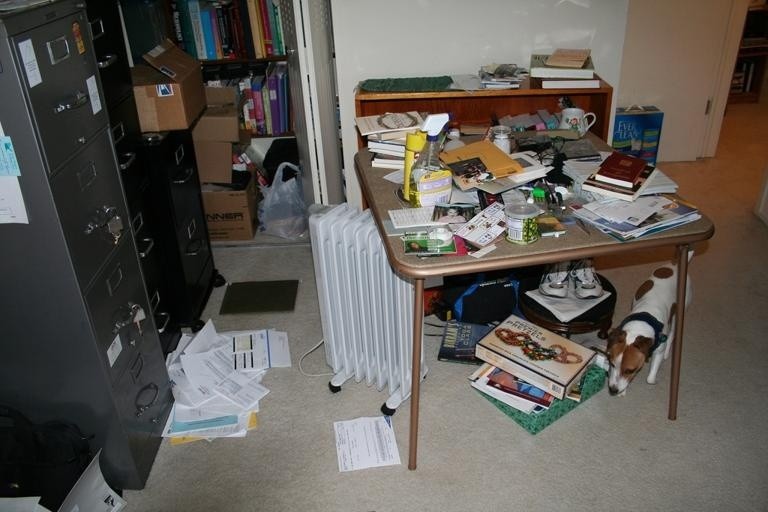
[2,0,328,491]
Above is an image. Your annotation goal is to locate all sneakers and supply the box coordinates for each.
[570,257,604,299]
[539,261,569,298]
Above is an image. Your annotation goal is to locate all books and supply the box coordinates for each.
[532,72,602,89]
[445,60,528,92]
[466,360,545,414]
[350,105,702,262]
[470,376,539,415]
[544,46,591,67]
[565,373,588,404]
[232,148,241,165]
[479,366,554,410]
[237,155,247,168]
[528,53,595,79]
[436,320,494,367]
[164,0,293,139]
[730,1,768,95]
[242,143,272,188]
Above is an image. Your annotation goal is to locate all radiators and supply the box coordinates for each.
[297,197,434,414]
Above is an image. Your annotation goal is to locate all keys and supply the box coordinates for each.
[113,231,121,244]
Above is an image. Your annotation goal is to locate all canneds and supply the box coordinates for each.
[490,126,511,154]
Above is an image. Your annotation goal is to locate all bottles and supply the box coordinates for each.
[492,125,517,155]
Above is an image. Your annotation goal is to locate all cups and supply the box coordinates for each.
[562,106,596,138]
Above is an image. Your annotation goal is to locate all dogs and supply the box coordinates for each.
[605,248,695,399]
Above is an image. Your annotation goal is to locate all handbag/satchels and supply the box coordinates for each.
[440,270,516,323]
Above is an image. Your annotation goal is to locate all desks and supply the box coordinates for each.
[352,127,717,465]
